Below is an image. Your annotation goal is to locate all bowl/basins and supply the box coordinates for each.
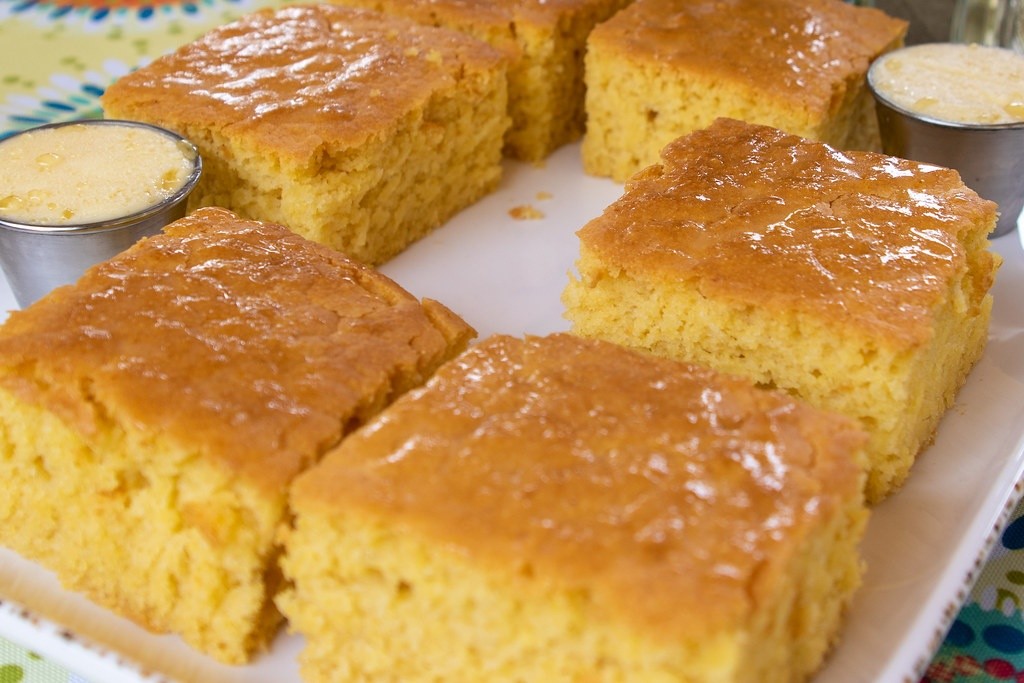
[864,39,1024,240]
[0,118,204,319]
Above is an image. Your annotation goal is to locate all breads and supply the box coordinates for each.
[581,0,911,184]
[98,3,509,271]
[1,204,478,665]
[329,0,632,163]
[272,330,874,683]
[561,114,1004,502]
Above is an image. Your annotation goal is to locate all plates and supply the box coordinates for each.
[0,0,1024,683]
